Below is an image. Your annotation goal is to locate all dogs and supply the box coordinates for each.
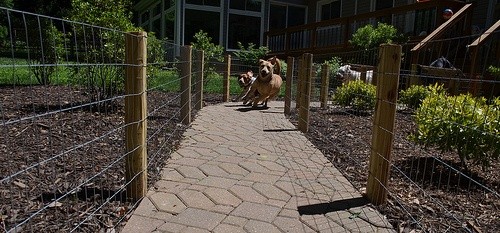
[336,64,373,85]
[232,56,282,109]
